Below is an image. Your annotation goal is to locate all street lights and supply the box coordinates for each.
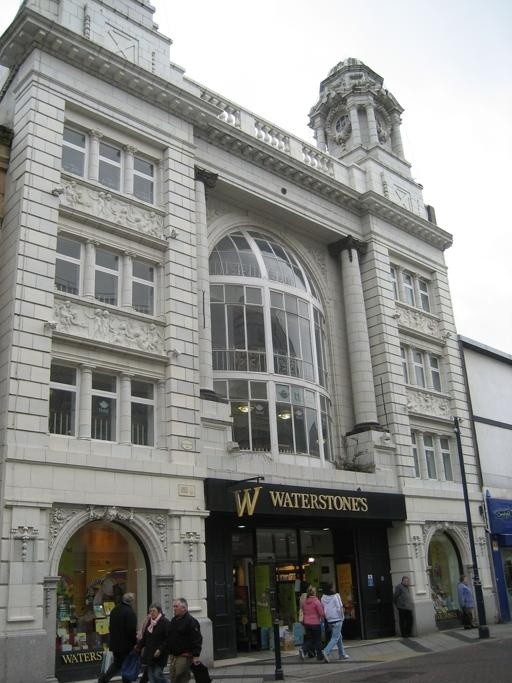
[449,412,490,641]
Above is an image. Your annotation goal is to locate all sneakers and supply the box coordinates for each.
[339,655,348,660]
[299,648,304,660]
[308,648,330,663]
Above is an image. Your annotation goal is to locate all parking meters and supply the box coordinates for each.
[268,585,286,681]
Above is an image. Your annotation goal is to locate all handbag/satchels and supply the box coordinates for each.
[298,609,303,623]
[100,650,115,675]
[190,661,211,683]
[121,650,142,681]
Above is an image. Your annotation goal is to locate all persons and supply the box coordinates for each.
[153,598,202,683]
[97,592,138,683]
[298,583,316,658]
[133,601,171,683]
[299,587,325,661]
[457,575,478,629]
[320,580,349,663]
[393,576,414,638]
